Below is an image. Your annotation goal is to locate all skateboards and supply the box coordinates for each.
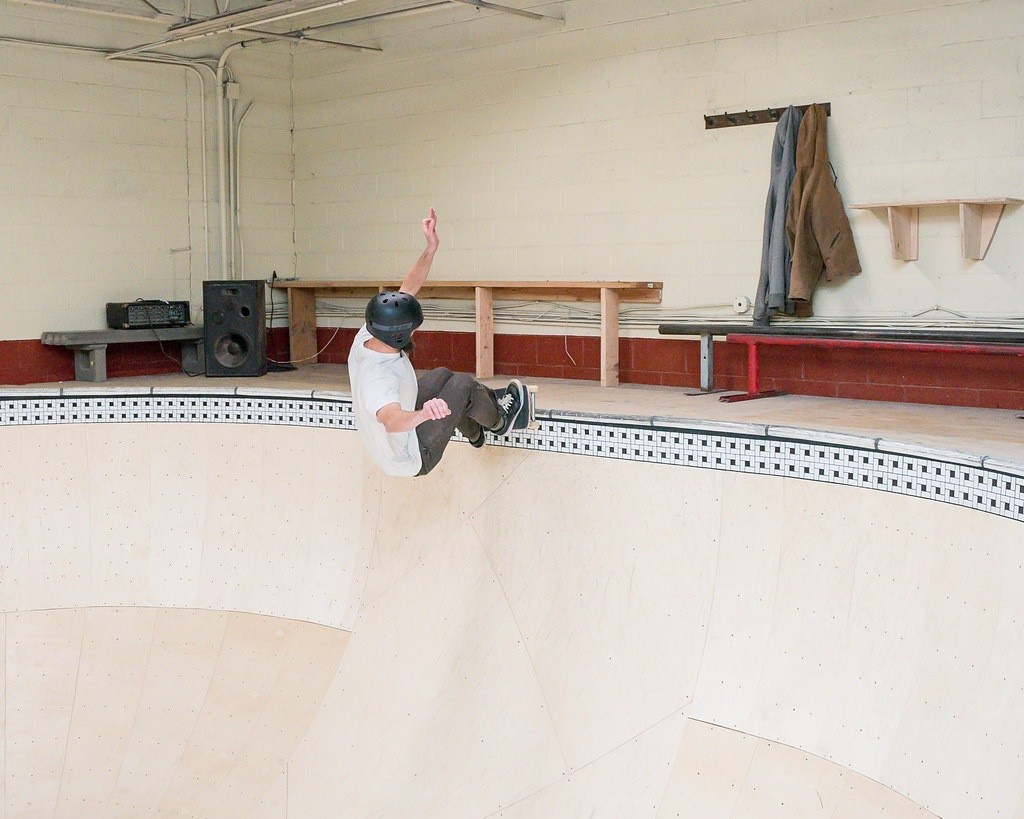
[487,385,539,430]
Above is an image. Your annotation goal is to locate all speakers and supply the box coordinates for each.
[203,279,268,378]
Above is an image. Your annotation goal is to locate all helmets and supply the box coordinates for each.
[365,290,423,348]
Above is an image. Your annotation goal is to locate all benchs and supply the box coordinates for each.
[272,276,666,385]
[44,322,210,381]
[653,318,1024,406]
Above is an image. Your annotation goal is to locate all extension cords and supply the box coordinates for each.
[267,277,296,283]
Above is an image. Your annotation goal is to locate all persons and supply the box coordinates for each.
[348,207,523,476]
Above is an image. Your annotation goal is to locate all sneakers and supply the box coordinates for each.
[468,426,486,449]
[490,379,523,438]
